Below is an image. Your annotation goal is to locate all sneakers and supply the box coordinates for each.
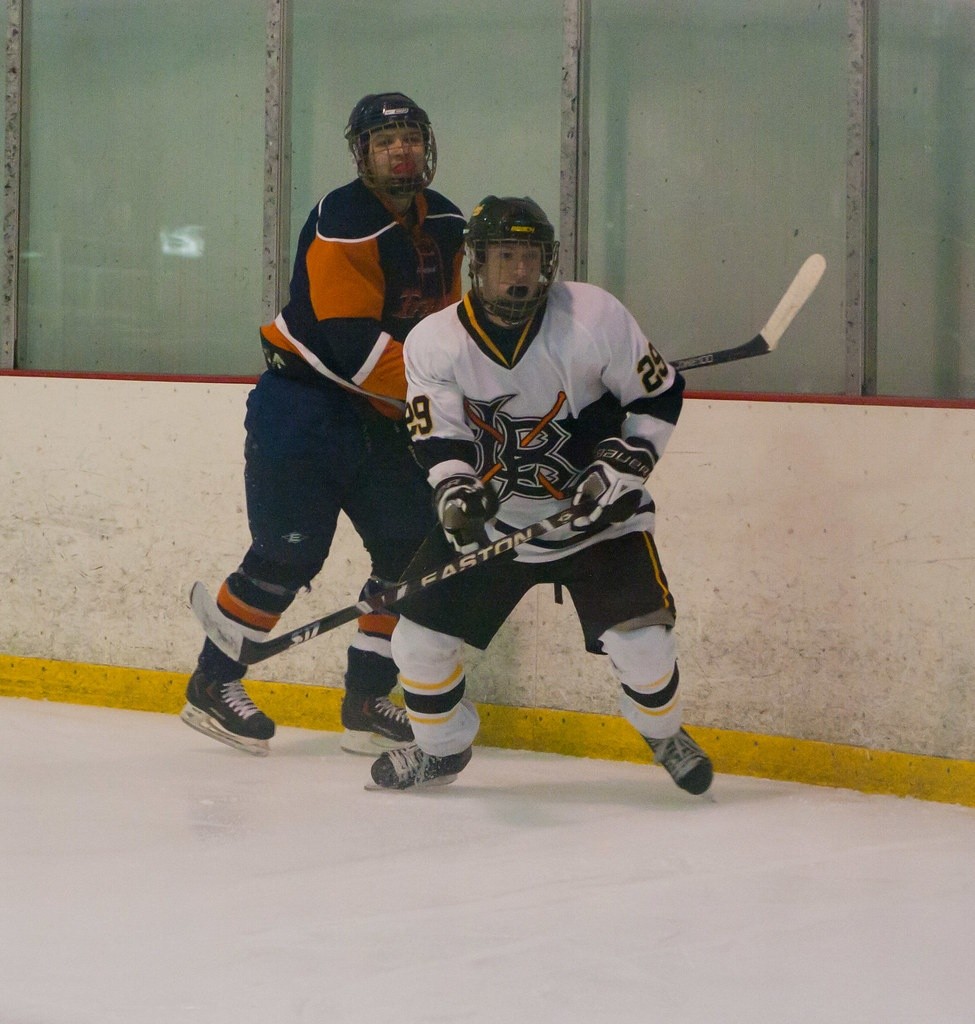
[180,665,275,757]
[363,739,472,793]
[339,672,419,757]
[641,726,713,796]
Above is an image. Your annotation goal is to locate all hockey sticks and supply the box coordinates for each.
[667,250,828,372]
[187,497,601,667]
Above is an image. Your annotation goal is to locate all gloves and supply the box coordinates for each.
[433,473,498,572]
[570,437,659,532]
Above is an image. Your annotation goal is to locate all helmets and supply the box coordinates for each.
[344,92,437,200]
[462,195,561,327]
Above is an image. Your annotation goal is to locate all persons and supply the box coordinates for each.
[178,93,469,761]
[363,196,713,802]
